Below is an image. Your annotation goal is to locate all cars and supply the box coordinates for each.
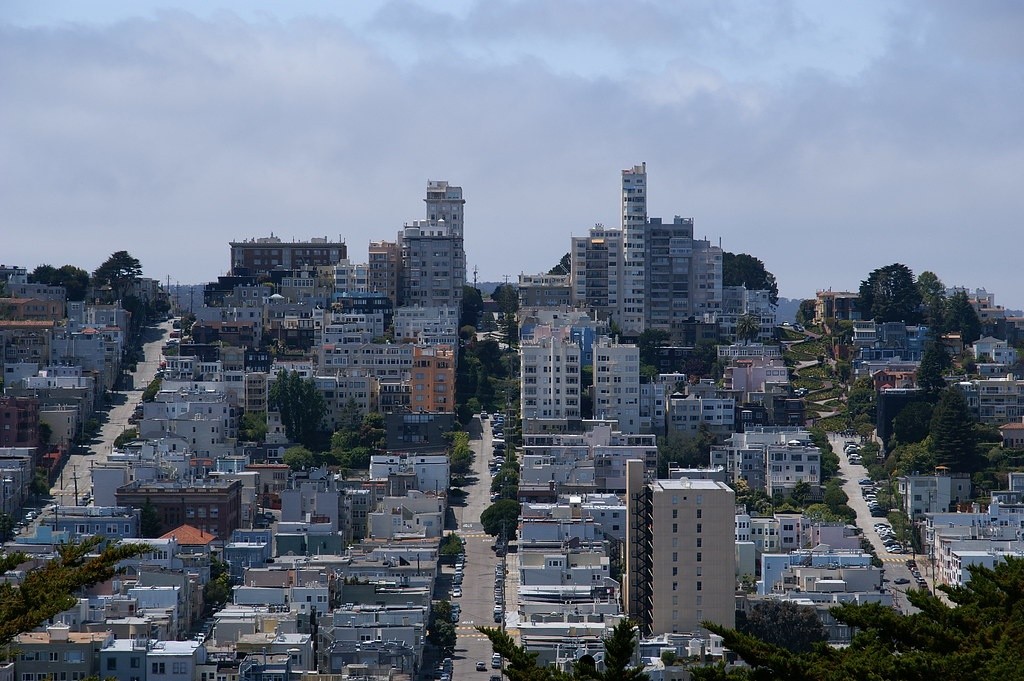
[843,440,863,464]
[11,511,36,534]
[261,519,269,526]
[450,553,465,622]
[496,549,504,557]
[490,675,501,681]
[906,561,929,590]
[481,408,489,419]
[460,538,467,545]
[78,495,90,506]
[489,410,506,503]
[874,522,913,553]
[859,479,875,485]
[476,662,488,671]
[265,511,274,519]
[435,658,454,681]
[494,563,503,622]
[861,485,883,517]
[491,652,502,669]
[894,577,910,584]
